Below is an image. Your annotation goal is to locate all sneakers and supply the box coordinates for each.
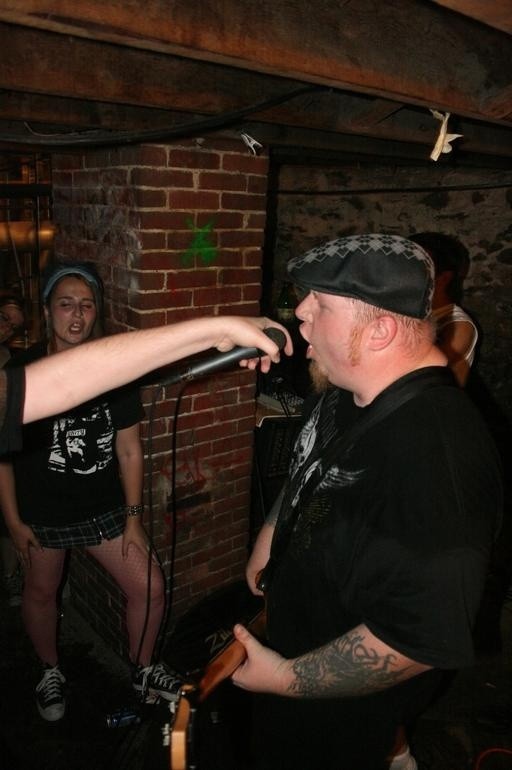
[34,664,68,723]
[130,662,186,704]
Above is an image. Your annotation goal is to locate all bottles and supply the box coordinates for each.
[276,278,299,328]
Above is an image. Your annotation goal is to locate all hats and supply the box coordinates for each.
[286,235,435,322]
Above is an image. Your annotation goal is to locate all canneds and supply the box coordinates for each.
[106,708,139,728]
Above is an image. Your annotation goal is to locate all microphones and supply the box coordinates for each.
[160,327,286,387]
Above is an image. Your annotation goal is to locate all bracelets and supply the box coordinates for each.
[125,502,143,517]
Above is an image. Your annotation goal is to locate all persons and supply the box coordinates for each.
[407,230,479,389]
[1,310,293,459]
[230,230,507,769]
[4,262,189,722]
[381,717,419,769]
[1,296,26,345]
